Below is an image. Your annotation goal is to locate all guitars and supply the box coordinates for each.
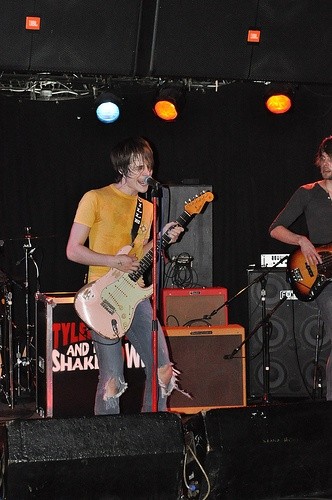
[70,190,213,341]
[287,241,332,302]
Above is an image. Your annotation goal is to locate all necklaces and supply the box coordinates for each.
[319,181,332,196]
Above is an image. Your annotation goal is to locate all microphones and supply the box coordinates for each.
[145,176,162,189]
[16,248,37,265]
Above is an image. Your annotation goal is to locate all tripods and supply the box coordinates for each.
[0,269,37,411]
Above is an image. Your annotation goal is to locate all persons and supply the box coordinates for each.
[66,136,184,416]
[268,135,332,401]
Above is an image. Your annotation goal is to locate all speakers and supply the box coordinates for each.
[161,287,228,326]
[237,269,332,397]
[0,411,185,500]
[162,323,247,413]
[182,401,332,500]
